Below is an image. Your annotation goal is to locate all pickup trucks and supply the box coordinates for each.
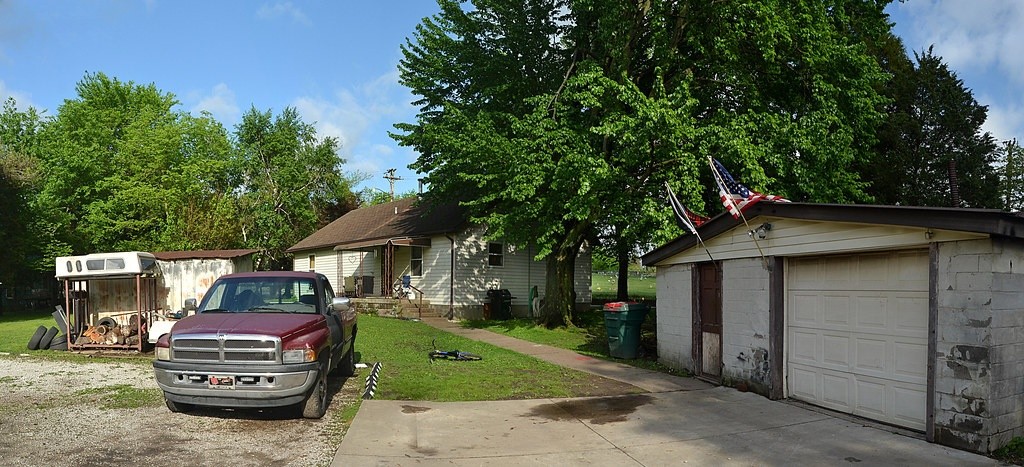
[153,270,359,419]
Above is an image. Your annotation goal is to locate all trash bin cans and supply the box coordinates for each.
[602,303,651,360]
[487,289,511,321]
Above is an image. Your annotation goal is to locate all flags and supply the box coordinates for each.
[709,157,791,220]
[666,188,710,233]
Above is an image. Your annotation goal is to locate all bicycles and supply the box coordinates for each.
[428,339,483,362]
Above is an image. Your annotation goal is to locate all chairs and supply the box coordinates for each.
[394,275,411,298]
[298,294,315,306]
[237,290,259,311]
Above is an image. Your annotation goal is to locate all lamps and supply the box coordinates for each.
[747,222,771,238]
